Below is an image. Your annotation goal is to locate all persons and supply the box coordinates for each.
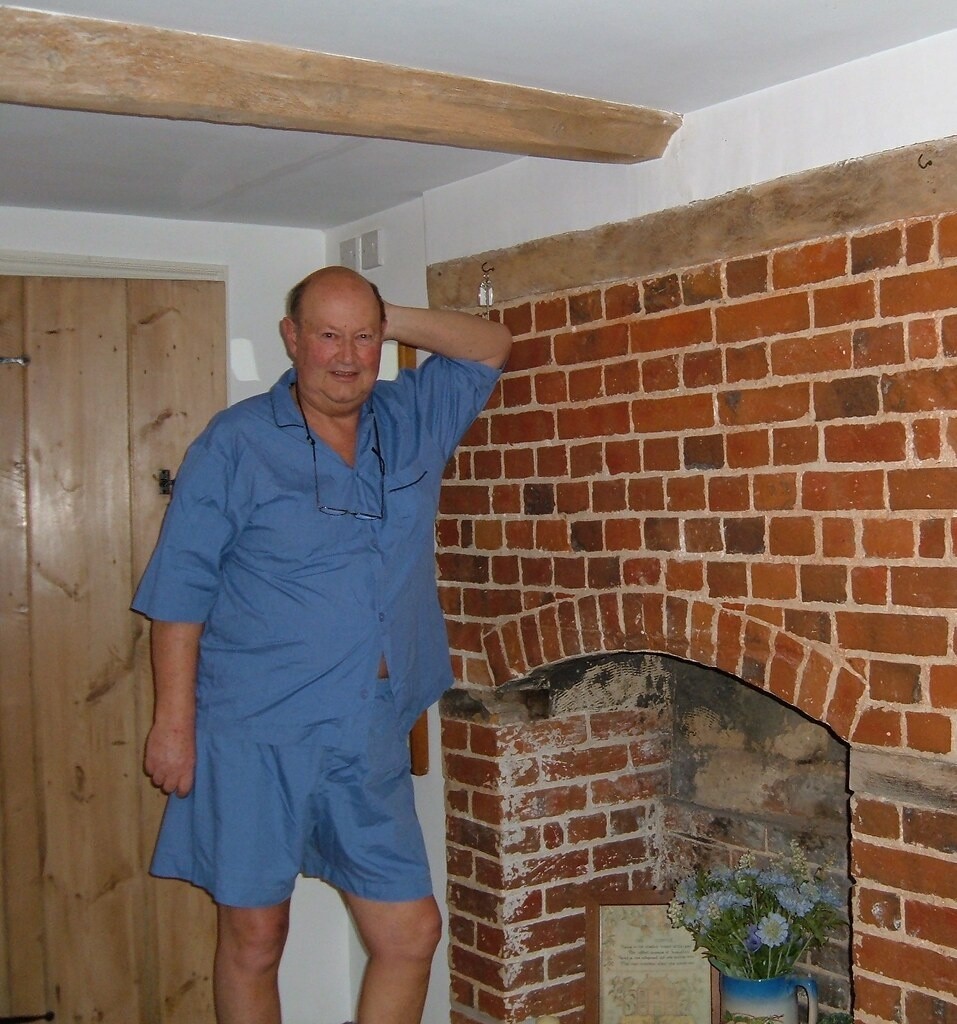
[128,265,512,1024]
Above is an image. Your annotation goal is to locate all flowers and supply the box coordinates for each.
[667,837,848,980]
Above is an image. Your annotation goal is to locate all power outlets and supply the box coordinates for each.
[340,236,361,274]
[360,229,384,270]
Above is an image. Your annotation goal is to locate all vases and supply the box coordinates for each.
[718,973,817,1024]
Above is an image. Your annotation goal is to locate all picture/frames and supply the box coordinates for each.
[584,889,721,1024]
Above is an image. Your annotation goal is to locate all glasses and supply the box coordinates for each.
[306,435,387,521]
